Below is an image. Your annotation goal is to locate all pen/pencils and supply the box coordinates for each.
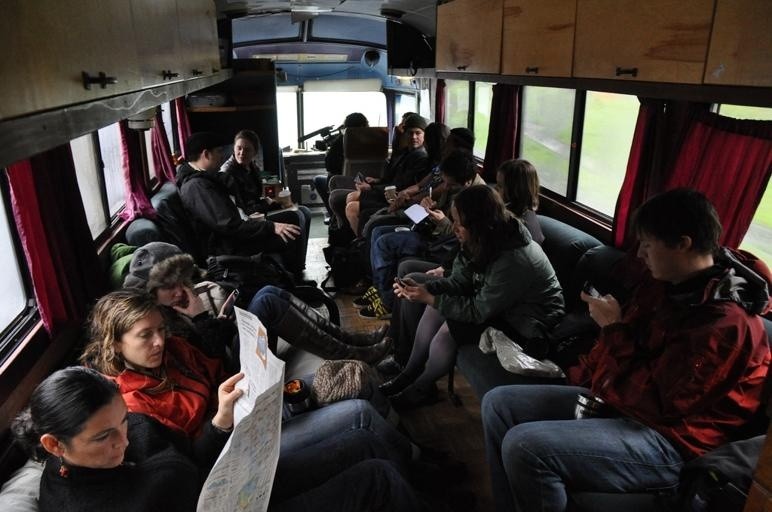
[430,186,433,200]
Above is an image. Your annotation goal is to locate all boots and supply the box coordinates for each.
[276,289,394,367]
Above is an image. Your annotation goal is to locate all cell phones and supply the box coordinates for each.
[394,277,405,287]
[582,281,602,299]
[223,289,240,318]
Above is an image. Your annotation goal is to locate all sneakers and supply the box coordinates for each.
[353,286,380,309]
[359,297,389,319]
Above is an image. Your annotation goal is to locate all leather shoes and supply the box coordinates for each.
[378,380,401,395]
[389,383,438,405]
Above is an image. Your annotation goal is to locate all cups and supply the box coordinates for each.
[283,378,313,416]
[572,392,609,420]
[384,185,398,201]
[279,191,293,207]
[249,212,265,223]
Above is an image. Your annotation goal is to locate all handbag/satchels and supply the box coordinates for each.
[333,245,370,287]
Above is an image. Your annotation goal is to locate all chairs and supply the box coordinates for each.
[449,214,597,408]
[342,127,389,179]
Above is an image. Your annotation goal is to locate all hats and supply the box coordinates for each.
[122,241,195,293]
[310,359,370,407]
[405,114,426,130]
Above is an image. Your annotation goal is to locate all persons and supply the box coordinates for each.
[481,186,771,511]
[312,113,564,409]
[12,131,474,510]
